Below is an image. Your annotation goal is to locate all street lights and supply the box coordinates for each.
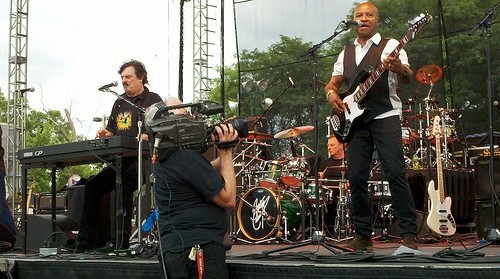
[92,115,111,128]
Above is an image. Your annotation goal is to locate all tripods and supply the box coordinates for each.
[260,28,355,256]
[106,88,155,257]
[463,1,500,253]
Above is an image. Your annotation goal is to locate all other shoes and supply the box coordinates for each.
[402,236,418,251]
[99,240,128,253]
[65,240,94,252]
[342,236,374,252]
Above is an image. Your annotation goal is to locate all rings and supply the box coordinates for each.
[333,105,335,108]
[388,63,391,68]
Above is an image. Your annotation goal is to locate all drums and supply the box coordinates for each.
[237,185,301,242]
[400,127,415,142]
[281,157,314,188]
[307,185,332,204]
[257,160,286,188]
[426,125,454,142]
[299,156,319,174]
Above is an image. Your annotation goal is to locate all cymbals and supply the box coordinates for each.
[238,131,272,140]
[274,125,314,139]
[416,64,443,85]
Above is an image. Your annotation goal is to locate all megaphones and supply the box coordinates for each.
[240,79,254,92]
[261,97,273,111]
[228,100,244,111]
[255,78,268,91]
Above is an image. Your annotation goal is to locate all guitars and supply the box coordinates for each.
[328,12,436,143]
[421,127,455,238]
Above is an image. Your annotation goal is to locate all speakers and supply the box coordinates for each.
[12,214,80,252]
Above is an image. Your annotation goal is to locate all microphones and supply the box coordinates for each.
[341,20,362,28]
[98,81,118,90]
[17,87,35,92]
[288,76,296,88]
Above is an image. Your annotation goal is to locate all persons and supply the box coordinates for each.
[65,59,162,253]
[145,97,240,279]
[278,133,349,240]
[324,2,421,254]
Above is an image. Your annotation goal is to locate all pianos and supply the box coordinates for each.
[14,136,148,252]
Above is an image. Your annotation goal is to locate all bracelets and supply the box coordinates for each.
[326,90,335,103]
[398,65,409,78]
